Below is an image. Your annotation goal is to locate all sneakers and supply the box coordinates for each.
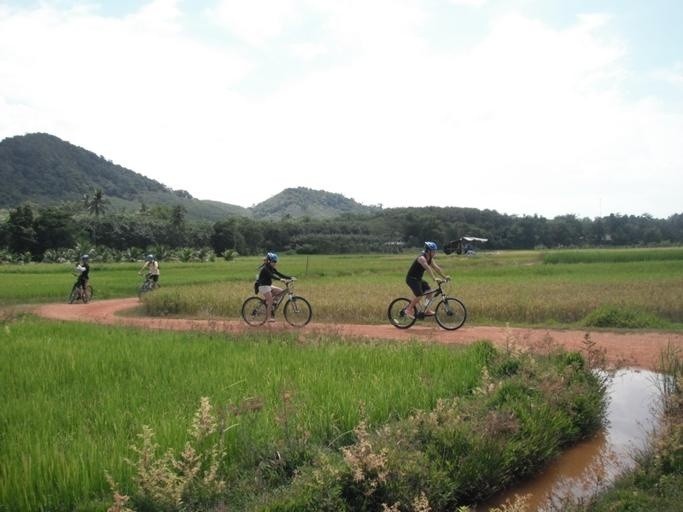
[404,307,416,320]
[424,309,436,316]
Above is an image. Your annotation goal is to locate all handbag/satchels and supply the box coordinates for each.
[254,279,260,294]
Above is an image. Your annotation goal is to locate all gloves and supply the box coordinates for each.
[434,277,442,284]
[445,275,452,282]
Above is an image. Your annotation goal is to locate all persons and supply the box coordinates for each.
[404,240,452,319]
[136,255,159,290]
[252,253,296,323]
[68,254,90,301]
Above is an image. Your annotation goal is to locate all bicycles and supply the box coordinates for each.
[388,275,467,329]
[67,273,92,303]
[241,278,312,328]
[136,272,161,302]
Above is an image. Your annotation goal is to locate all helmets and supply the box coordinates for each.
[267,252,278,263]
[424,239,439,252]
